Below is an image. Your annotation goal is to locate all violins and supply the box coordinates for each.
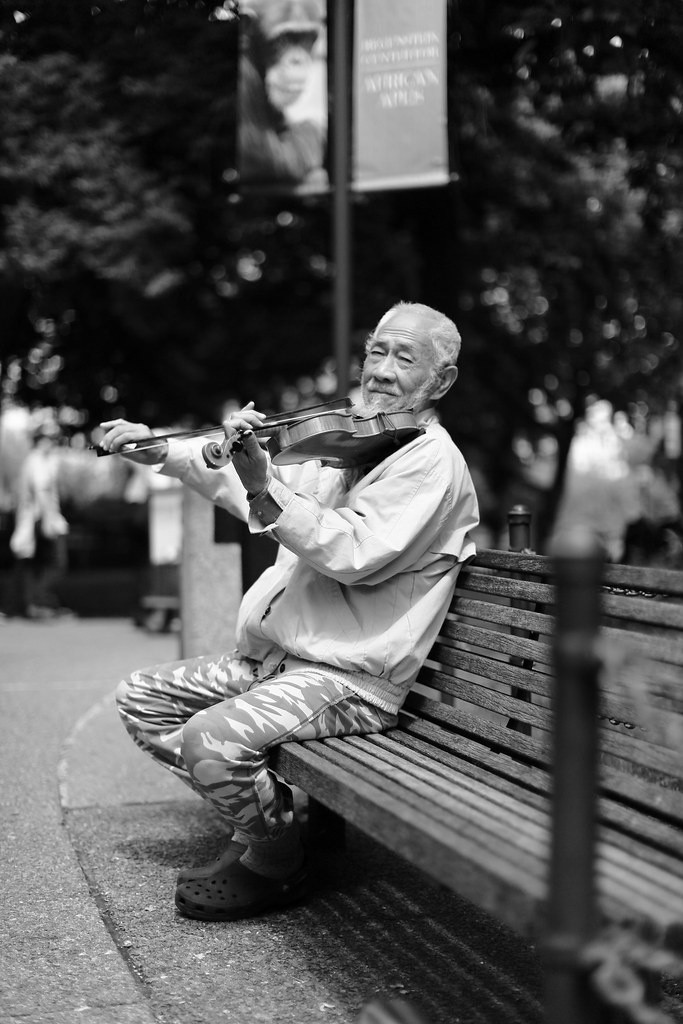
[202,407,422,471]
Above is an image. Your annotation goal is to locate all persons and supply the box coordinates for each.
[99,302,479,921]
[11,423,75,620]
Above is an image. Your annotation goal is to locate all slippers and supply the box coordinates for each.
[177,838,249,885]
[174,857,316,922]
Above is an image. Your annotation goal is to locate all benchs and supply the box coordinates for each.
[267,546,683,971]
